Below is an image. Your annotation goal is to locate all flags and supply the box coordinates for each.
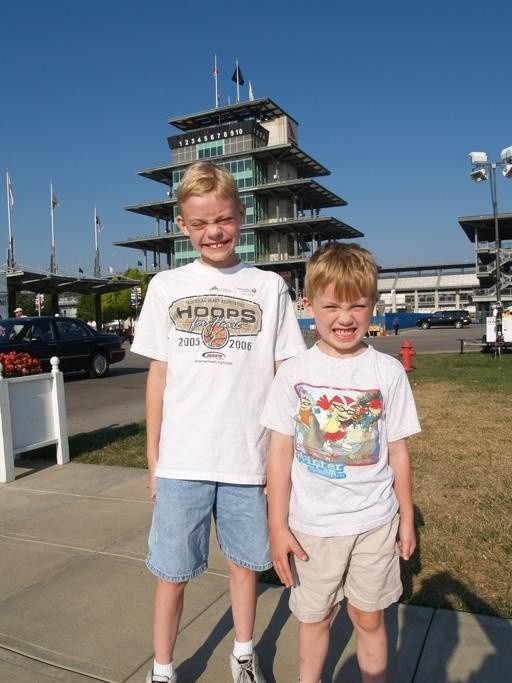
[79,266,84,274]
[231,64,244,86]
[138,260,142,266]
[213,66,219,79]
[8,176,15,208]
[96,217,103,232]
[55,193,59,209]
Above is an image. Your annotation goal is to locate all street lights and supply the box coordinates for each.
[469,146,512,359]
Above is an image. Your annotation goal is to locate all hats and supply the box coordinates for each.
[14,308,22,312]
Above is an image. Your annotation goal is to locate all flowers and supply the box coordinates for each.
[1,348,44,380]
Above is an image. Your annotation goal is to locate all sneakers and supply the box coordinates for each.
[228,652,265,683]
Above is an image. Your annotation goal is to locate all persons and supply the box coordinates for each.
[127,155,308,683]
[130,314,139,342]
[122,315,133,345]
[491,305,498,316]
[87,316,97,333]
[259,241,421,681]
[392,314,400,335]
[13,306,29,335]
[101,315,126,336]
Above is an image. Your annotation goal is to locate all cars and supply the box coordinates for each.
[0,316,126,378]
[416,310,471,329]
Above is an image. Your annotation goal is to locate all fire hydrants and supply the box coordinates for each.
[399,338,416,372]
